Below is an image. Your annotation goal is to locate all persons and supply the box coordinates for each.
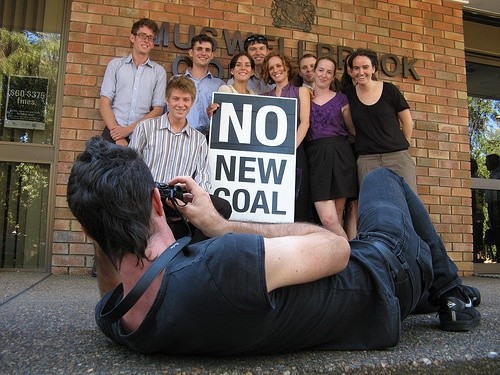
[66,136,481,357]
[340,58,377,241]
[127,76,212,193]
[164,35,227,137]
[227,34,277,94]
[299,53,318,93]
[470,154,500,263]
[100,18,167,146]
[342,49,419,199]
[206,52,261,118]
[258,50,310,221]
[303,56,356,241]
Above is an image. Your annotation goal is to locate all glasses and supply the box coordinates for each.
[245,36,267,50]
[130,32,156,41]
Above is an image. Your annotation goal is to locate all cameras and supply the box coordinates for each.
[155,182,184,219]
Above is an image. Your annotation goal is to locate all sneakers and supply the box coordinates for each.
[435,285,481,331]
[461,285,481,307]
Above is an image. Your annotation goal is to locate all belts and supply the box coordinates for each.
[368,240,408,321]
